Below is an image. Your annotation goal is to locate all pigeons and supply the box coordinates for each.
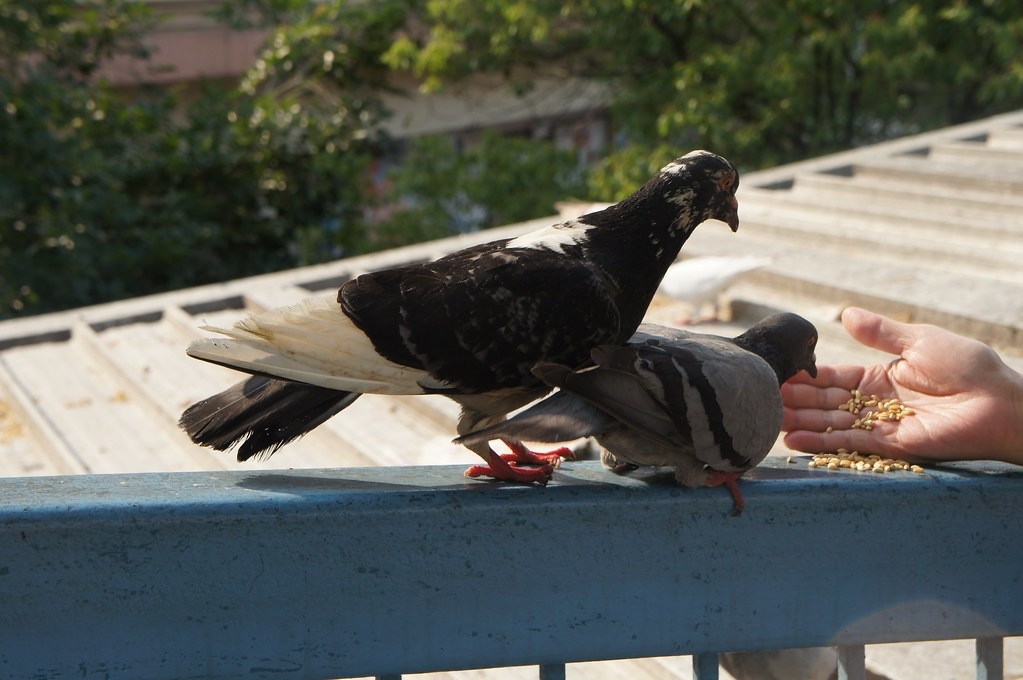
[179,151,817,507]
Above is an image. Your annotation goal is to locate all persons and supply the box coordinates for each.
[779,307,1022,466]
